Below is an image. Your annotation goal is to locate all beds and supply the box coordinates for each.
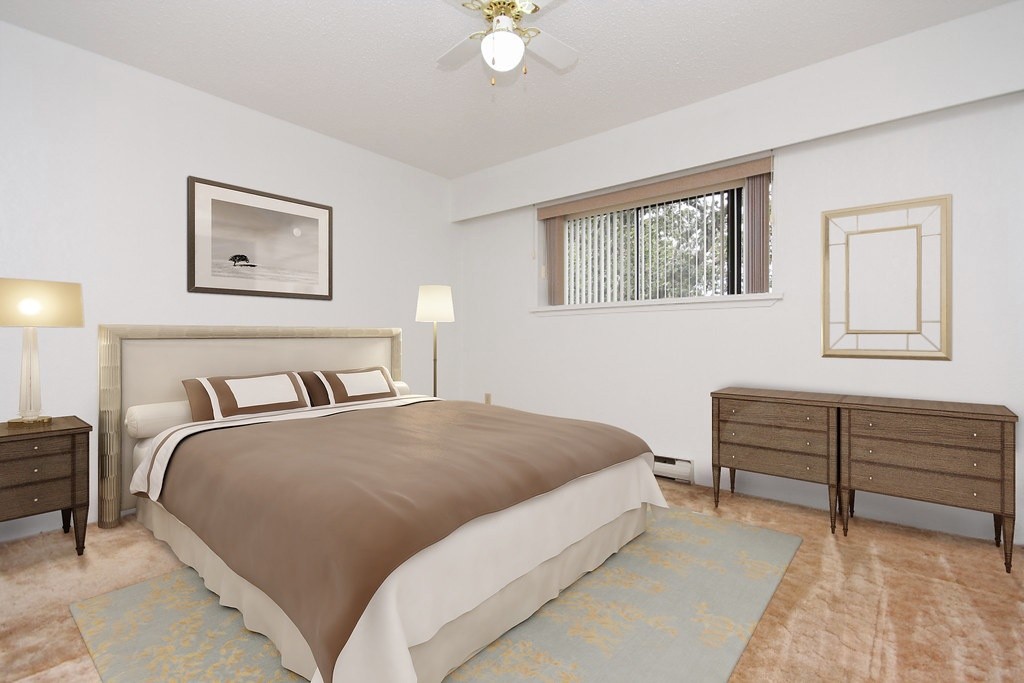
[97,322,670,682]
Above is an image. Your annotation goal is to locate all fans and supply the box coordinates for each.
[436,0,581,72]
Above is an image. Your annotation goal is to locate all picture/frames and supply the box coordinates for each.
[187,176,333,301]
[821,193,953,361]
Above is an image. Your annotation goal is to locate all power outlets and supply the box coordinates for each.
[484,392,492,405]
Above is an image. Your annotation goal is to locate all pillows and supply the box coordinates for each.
[298,365,401,407]
[181,369,312,422]
[123,379,410,439]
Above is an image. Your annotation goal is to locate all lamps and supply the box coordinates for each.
[415,285,456,397]
[0,276,85,430]
[461,0,541,85]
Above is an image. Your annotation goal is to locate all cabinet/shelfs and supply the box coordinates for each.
[837,394,1018,574]
[710,386,847,535]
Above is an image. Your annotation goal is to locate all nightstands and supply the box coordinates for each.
[0,415,94,556]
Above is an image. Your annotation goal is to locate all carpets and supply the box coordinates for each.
[69,503,803,683]
